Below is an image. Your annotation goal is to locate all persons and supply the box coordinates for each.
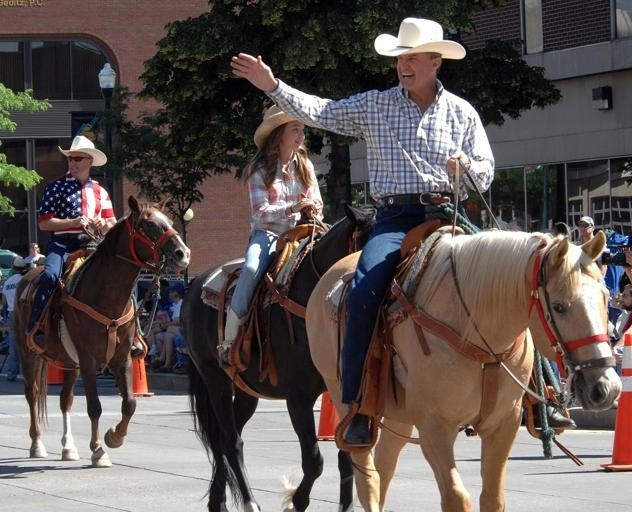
[491,210,632,407]
[221,105,323,363]
[2,257,25,382]
[25,243,45,268]
[26,136,143,355]
[231,17,578,446]
[144,281,189,373]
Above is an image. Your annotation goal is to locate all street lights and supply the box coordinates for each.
[98,63,119,212]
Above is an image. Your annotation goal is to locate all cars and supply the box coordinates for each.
[0,247,25,295]
[135,266,195,304]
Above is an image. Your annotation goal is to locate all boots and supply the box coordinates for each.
[218,306,250,370]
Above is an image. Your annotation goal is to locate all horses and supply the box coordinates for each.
[304,227,623,512]
[179,202,377,512]
[12,194,192,468]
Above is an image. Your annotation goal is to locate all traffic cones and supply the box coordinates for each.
[595,332,632,472]
[132,334,155,397]
[45,354,64,387]
[314,391,342,442]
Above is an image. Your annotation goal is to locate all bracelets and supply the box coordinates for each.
[290,205,294,213]
[463,160,471,173]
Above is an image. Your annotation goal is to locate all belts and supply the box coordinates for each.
[383,191,457,206]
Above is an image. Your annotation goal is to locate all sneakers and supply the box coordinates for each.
[128,336,144,358]
[26,329,47,355]
[6,370,16,381]
[148,345,188,374]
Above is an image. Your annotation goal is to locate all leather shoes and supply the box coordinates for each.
[343,413,373,448]
[521,401,579,431]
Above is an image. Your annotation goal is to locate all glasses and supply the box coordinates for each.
[67,157,91,162]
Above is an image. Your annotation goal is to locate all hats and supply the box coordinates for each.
[57,134,108,168]
[12,257,26,268]
[373,15,468,61]
[578,215,595,225]
[252,104,308,148]
[165,282,185,298]
